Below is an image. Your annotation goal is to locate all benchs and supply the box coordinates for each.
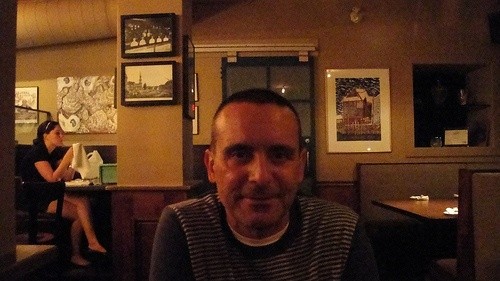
[459,169,500,281]
[357,162,500,228]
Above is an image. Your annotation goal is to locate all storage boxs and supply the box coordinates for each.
[99,163,117,183]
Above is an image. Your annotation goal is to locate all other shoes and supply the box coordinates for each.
[86,247,113,258]
[68,258,96,270]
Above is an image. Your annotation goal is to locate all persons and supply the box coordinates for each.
[149,88,380,281]
[21,119,109,268]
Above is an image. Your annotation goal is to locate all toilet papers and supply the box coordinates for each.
[71,142,90,179]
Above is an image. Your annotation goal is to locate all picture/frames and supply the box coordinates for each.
[325,69,392,153]
[15,87,39,123]
[120,13,177,58]
[184,36,195,119]
[121,61,177,105]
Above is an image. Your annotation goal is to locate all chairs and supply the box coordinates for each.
[20,182,73,245]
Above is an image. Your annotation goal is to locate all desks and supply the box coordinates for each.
[65,178,117,191]
[372,198,459,226]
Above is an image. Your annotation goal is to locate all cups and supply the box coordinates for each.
[431,136,442,147]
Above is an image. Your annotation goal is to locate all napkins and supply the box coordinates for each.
[88,150,103,178]
[71,143,90,180]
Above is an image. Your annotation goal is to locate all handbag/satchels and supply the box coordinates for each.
[68,148,104,181]
